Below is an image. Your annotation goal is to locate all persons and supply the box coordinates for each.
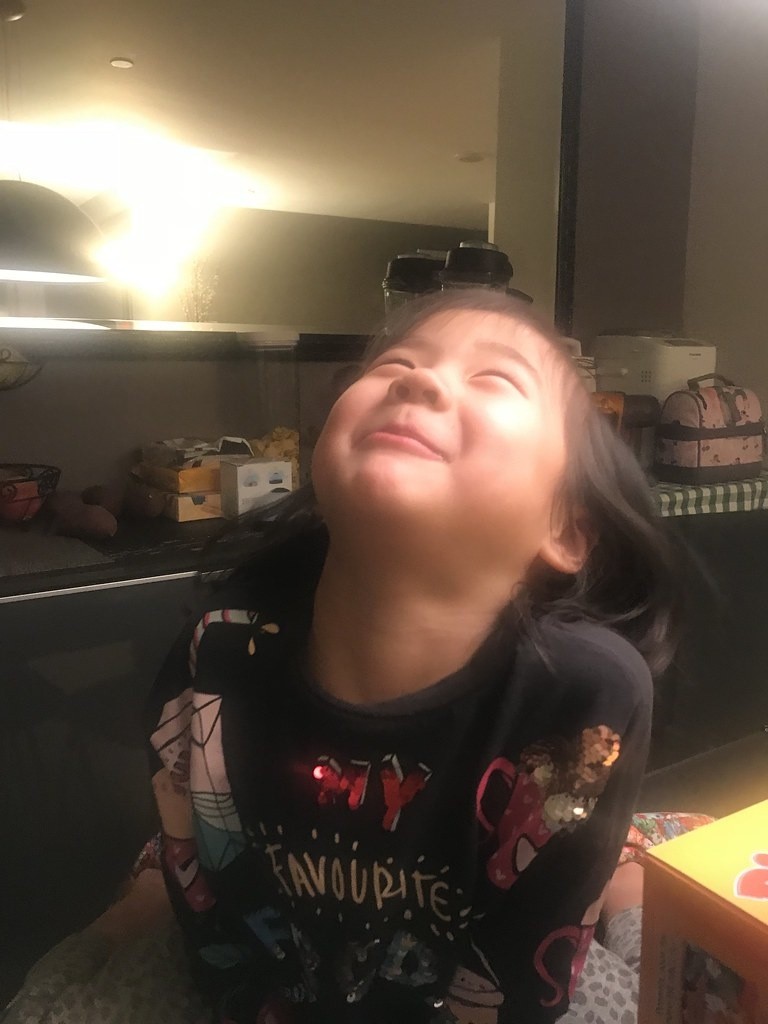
[0,280,743,1022]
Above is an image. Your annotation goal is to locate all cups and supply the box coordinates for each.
[620,395,659,488]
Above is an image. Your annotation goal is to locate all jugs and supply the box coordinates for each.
[382,248,447,316]
[438,241,534,306]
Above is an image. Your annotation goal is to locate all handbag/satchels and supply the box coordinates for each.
[654,374,763,483]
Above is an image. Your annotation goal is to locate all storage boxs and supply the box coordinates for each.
[127,482,222,523]
[636,798,768,1024]
[217,456,292,521]
[129,461,220,492]
